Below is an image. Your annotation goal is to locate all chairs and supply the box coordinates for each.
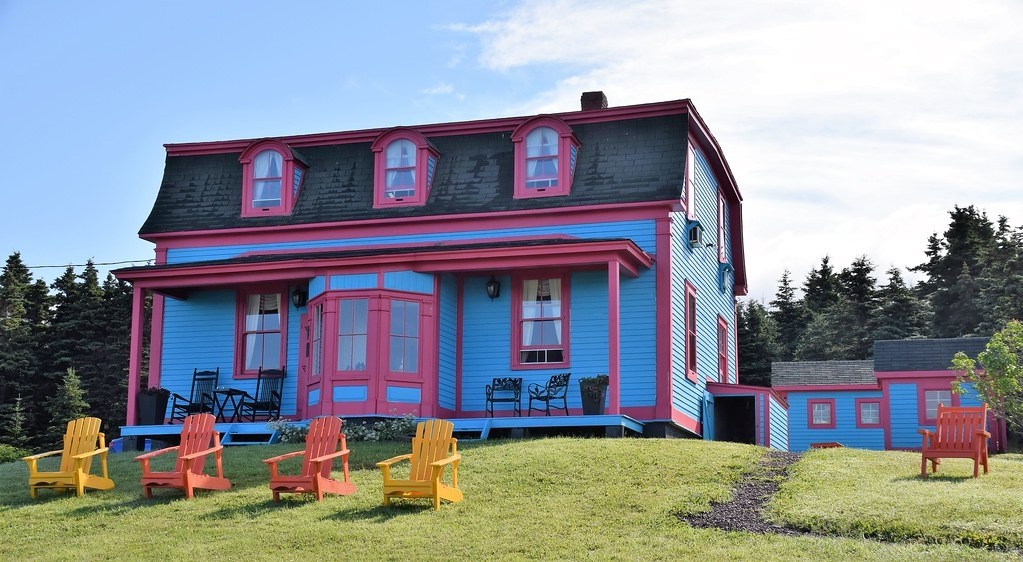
[528,372,572,417]
[917,403,992,479]
[239,365,285,423]
[485,374,523,418]
[168,365,219,423]
[377,420,464,511]
[133,414,232,502]
[263,416,356,502]
[27,416,117,500]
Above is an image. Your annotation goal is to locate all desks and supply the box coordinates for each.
[209,388,246,423]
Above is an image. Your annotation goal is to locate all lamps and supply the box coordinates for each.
[292,284,310,311]
[484,276,499,301]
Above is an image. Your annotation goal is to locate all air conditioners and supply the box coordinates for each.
[690,226,703,248]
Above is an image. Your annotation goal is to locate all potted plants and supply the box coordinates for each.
[140,387,168,425]
[579,373,608,415]
